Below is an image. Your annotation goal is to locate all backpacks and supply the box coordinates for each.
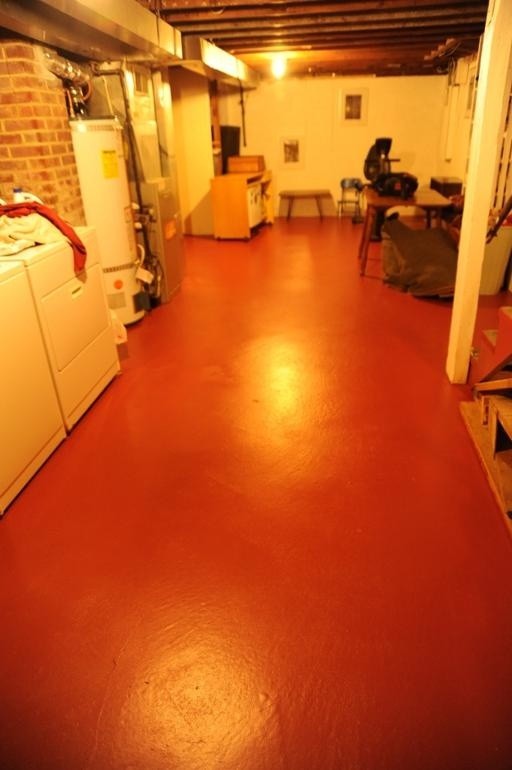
[367,172,419,201]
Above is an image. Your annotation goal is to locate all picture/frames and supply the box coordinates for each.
[340,89,368,124]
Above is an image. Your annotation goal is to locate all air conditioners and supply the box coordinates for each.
[69,115,152,325]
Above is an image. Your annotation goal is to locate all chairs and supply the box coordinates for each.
[337,178,363,218]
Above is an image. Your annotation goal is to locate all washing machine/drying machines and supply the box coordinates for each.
[0,259,68,517]
[6,224,124,433]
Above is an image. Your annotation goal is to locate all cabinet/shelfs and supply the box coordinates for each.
[0,226,122,518]
[431,177,462,197]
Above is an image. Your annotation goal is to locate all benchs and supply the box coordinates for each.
[280,189,330,222]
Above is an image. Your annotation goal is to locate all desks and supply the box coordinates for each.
[210,169,274,239]
[358,188,452,276]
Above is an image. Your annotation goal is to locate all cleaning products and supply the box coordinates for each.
[109,308,130,360]
[12,185,43,205]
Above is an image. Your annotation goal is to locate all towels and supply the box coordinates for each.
[0,191,87,273]
